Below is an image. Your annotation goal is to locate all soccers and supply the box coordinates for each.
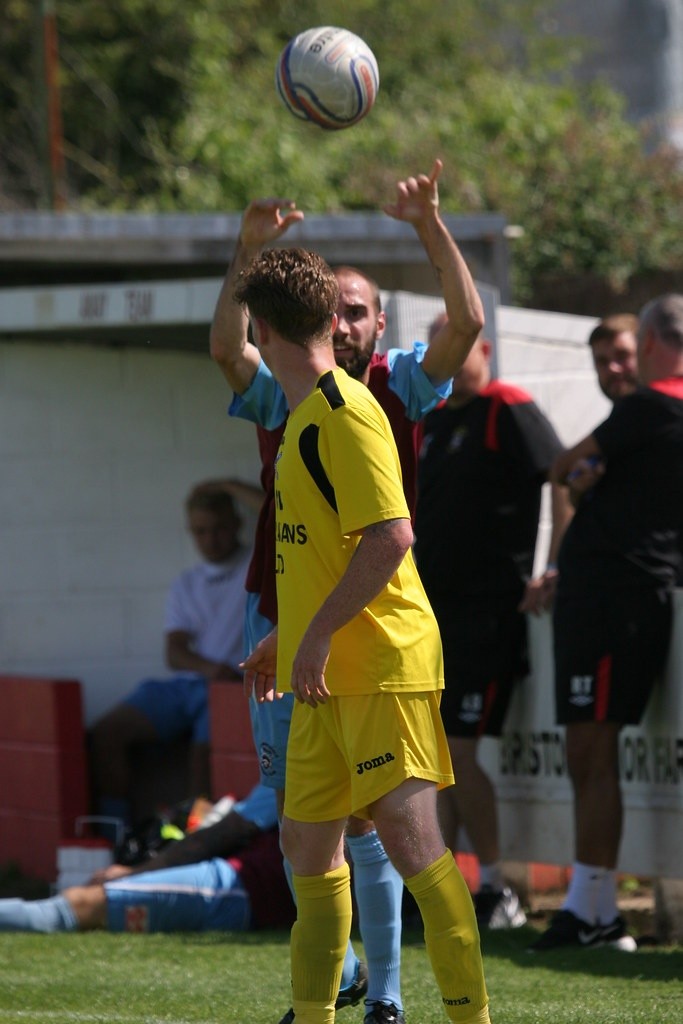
[276,26,380,131]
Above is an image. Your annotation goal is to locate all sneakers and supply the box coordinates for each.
[274,959,367,1024]
[358,1002,405,1024]
[523,906,628,953]
[471,883,527,931]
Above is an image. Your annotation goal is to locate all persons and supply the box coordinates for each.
[0,154,683,1024]
